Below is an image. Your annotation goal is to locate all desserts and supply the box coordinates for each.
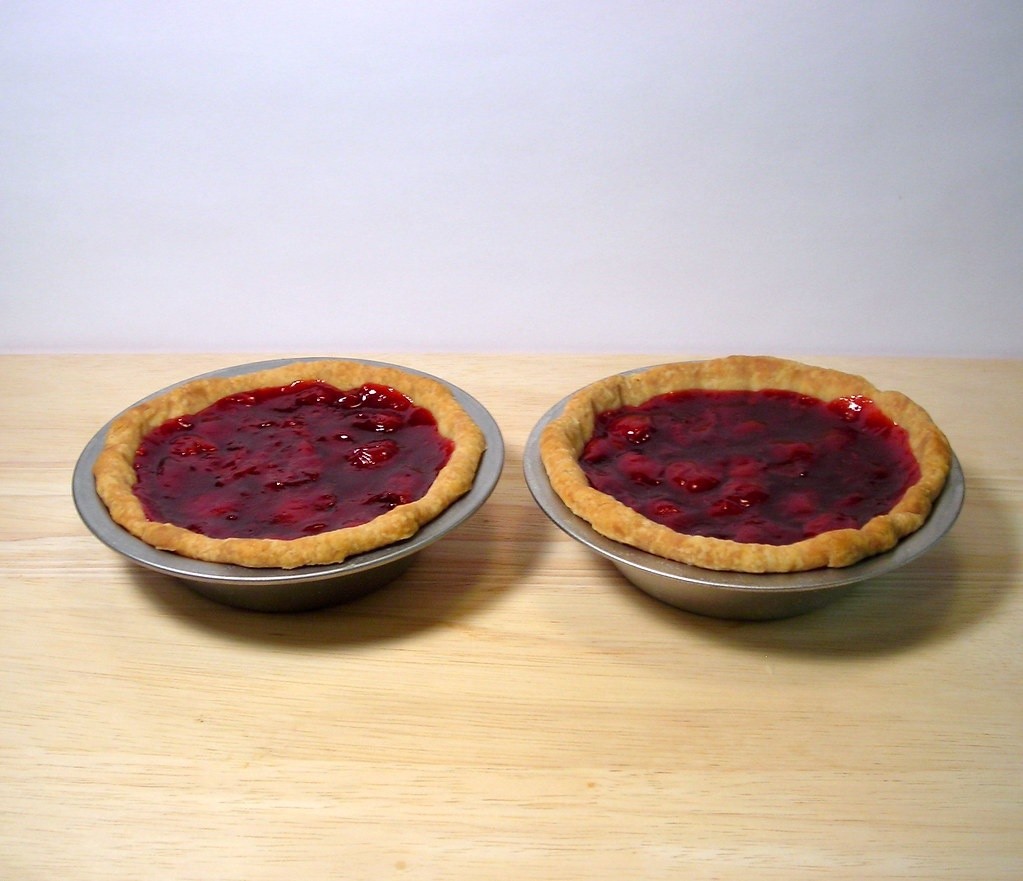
[537,354,952,576]
[92,359,486,570]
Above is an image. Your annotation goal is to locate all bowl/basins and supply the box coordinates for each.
[522,358,965,624]
[72,357,506,616]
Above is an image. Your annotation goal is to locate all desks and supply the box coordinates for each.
[1,352,1023,881]
